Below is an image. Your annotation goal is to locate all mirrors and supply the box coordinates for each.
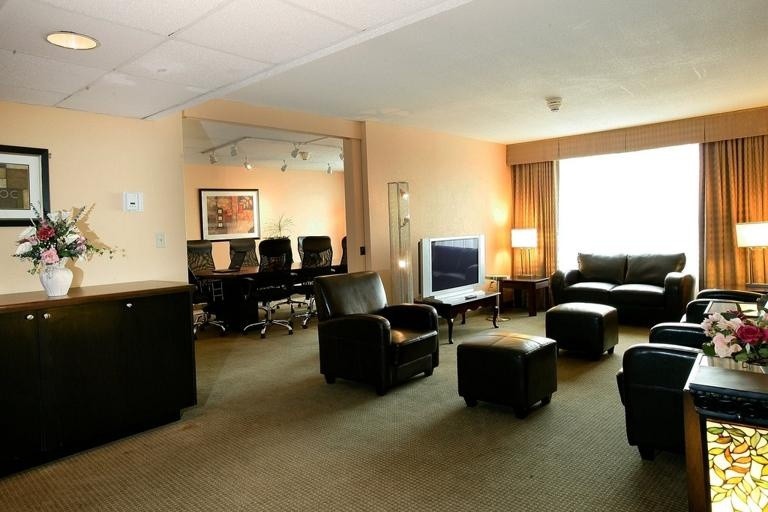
[387,180,414,305]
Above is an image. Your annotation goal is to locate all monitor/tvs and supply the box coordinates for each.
[418,234,485,303]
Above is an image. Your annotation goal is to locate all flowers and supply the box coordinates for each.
[698,305,768,369]
[7,199,129,281]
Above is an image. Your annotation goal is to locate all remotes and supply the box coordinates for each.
[465,295,477,299]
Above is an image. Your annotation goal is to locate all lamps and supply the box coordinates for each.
[388,181,412,303]
[734,220,768,289]
[510,228,539,280]
[200,134,345,175]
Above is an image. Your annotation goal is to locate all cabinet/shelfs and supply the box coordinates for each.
[681,352,767,512]
[0,277,202,481]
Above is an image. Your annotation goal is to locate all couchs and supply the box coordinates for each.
[549,250,697,330]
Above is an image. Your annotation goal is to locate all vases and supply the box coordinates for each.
[39,257,75,298]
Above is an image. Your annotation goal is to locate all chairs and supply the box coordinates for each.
[615,320,714,473]
[187,235,347,341]
[679,288,768,324]
[313,268,442,399]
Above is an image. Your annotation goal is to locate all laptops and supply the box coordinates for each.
[212,251,246,273]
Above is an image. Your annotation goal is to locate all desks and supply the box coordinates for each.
[702,299,767,320]
[414,290,502,345]
[484,275,510,322]
[498,274,556,317]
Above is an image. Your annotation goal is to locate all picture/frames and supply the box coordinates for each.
[197,187,262,243]
[0,143,51,230]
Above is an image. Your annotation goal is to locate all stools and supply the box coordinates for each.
[544,301,621,363]
[456,329,561,421]
